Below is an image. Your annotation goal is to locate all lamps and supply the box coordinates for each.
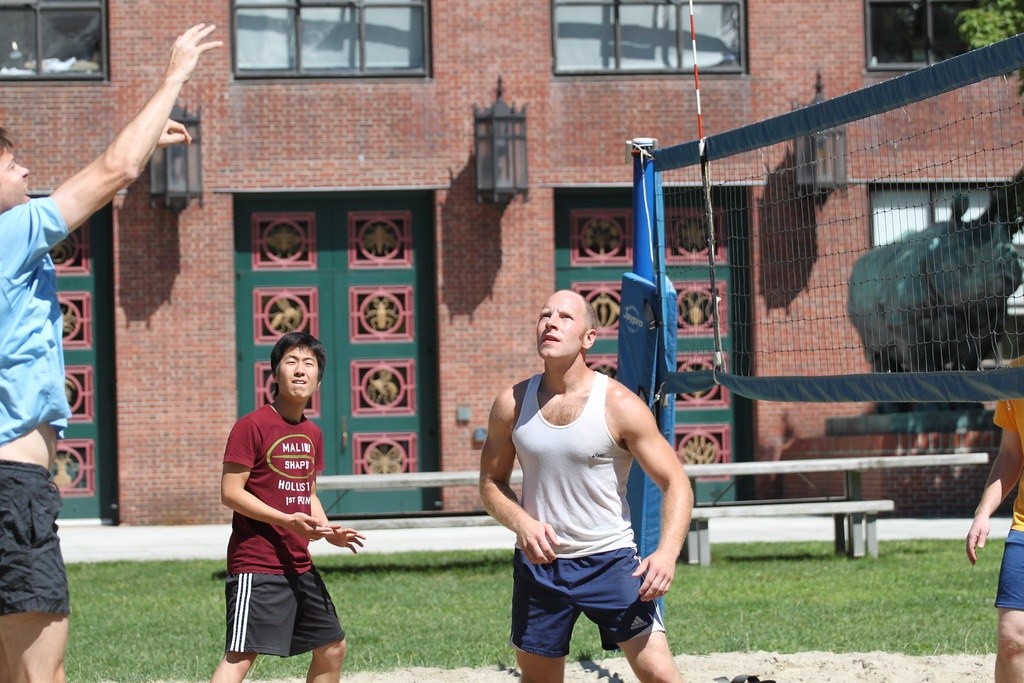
[471,74,533,206]
[147,103,204,213]
[791,69,852,210]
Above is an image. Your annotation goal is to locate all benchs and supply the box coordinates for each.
[689,493,901,561]
[317,515,516,534]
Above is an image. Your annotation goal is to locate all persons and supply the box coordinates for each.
[208,332,366,682]
[478,290,696,681]
[966,285,1024,683]
[2,21,225,680]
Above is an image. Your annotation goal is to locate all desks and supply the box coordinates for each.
[314,449,996,496]
